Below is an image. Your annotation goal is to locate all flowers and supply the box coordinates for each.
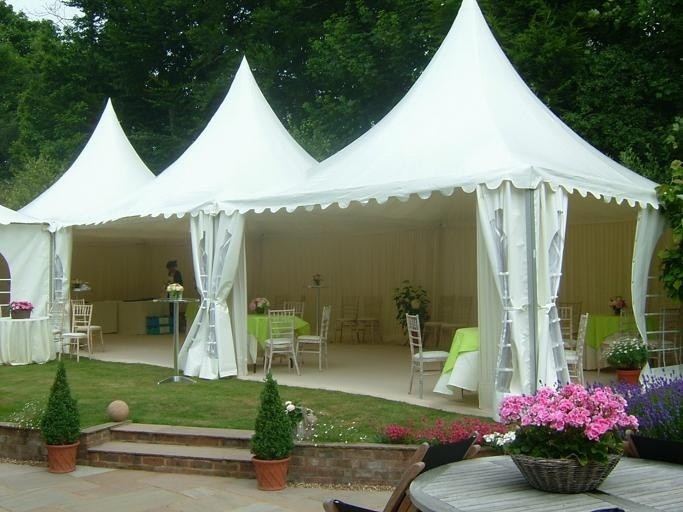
[494,380,642,465]
[164,282,185,302]
[10,300,34,310]
[606,294,629,315]
[248,297,271,315]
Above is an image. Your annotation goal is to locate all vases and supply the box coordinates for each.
[168,292,183,302]
[10,309,31,319]
[256,307,264,314]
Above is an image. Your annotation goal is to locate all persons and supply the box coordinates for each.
[165,259,183,317]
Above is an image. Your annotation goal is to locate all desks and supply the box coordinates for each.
[146,297,205,387]
[409,453,683,512]
[118,298,171,338]
[88,300,117,335]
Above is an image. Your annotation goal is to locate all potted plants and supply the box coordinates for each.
[40,358,80,474]
[389,278,433,351]
[250,366,294,495]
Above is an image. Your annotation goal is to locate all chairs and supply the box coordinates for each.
[322,429,482,512]
[555,304,682,386]
[404,312,453,400]
[42,298,104,365]
[262,294,381,377]
